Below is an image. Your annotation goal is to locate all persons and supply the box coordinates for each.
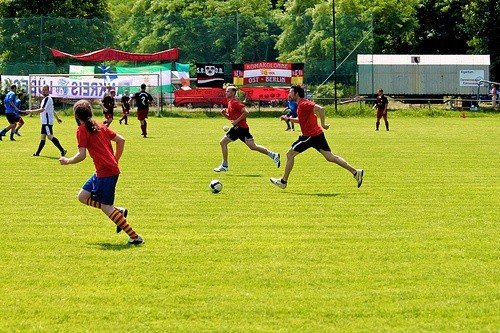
[103,85,112,104]
[214,86,281,172]
[285,94,299,132]
[0,85,19,141]
[128,83,154,138]
[14,93,24,136]
[371,89,390,131]
[60,100,145,245]
[119,90,130,125]
[270,86,363,189]
[25,85,67,158]
[491,84,496,107]
[100,91,117,127]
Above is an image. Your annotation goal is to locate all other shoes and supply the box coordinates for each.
[61,149,67,157]
[125,123,128,125]
[32,152,39,156]
[285,127,295,131]
[140,132,147,137]
[119,120,121,124]
[15,130,21,136]
[10,137,16,141]
[0,132,3,141]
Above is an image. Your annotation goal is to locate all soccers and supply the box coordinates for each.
[224,126,229,132]
[209,180,222,193]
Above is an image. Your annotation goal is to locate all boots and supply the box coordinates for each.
[375,122,380,131]
[385,121,389,131]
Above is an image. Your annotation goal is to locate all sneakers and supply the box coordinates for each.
[270,177,287,189]
[354,169,363,189]
[213,165,228,172]
[273,153,280,169]
[116,206,128,233]
[123,235,145,248]
